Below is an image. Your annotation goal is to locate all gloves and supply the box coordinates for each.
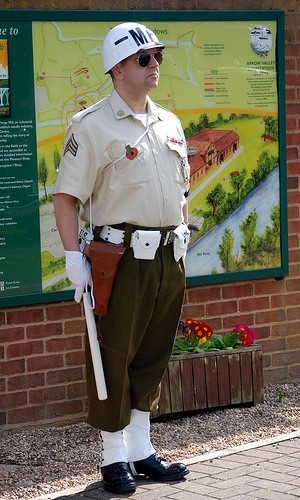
[65,251,92,302]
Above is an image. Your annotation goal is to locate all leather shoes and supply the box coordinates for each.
[100,461,137,493]
[128,452,190,482]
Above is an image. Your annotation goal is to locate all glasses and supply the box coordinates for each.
[126,52,163,67]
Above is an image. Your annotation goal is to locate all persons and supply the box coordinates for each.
[51,21,191,495]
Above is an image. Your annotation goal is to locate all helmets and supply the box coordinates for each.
[101,22,165,75]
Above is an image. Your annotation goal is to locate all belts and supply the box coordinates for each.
[88,223,190,263]
